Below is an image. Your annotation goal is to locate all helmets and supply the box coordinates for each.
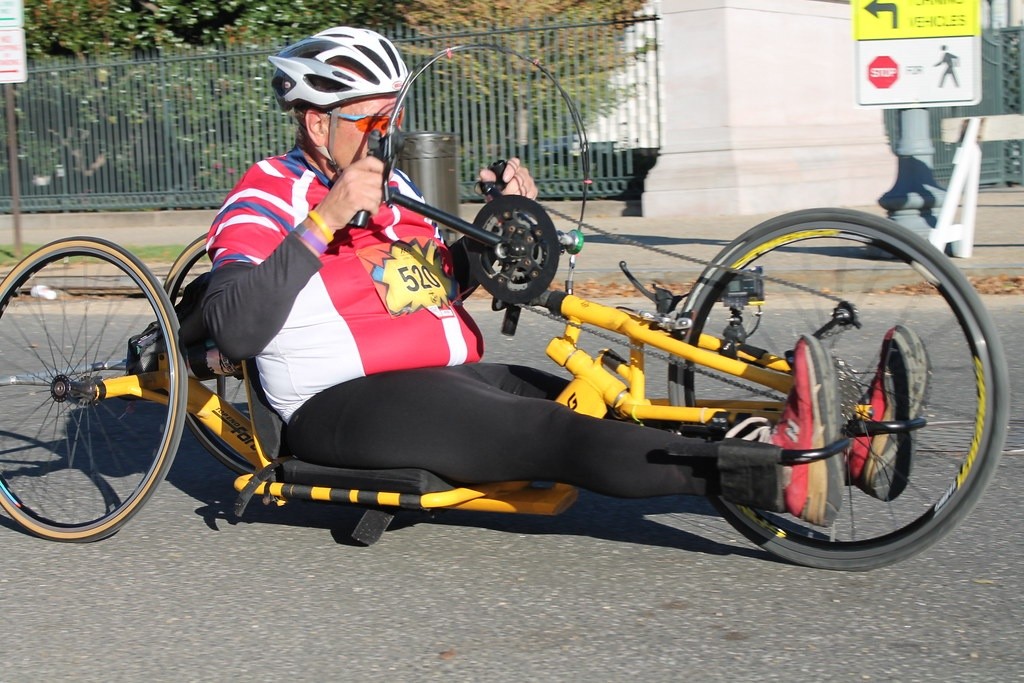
[267,26,411,112]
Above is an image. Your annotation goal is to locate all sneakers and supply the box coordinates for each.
[770,332,845,528]
[846,325,933,502]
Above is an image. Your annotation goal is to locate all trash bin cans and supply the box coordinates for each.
[390,132,461,246]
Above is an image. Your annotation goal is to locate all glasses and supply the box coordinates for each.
[326,105,405,136]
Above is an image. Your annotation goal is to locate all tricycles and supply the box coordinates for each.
[1,45,1012,574]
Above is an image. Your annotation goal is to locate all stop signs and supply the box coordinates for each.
[868,55,899,88]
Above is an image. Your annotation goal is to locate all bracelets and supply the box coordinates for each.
[308,210,334,243]
[294,223,328,255]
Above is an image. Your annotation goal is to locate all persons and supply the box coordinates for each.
[199,26,934,527]
